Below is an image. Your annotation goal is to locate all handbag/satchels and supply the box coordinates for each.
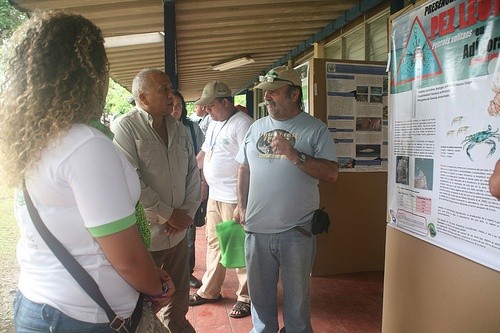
[195,199,207,227]
[215,220,246,268]
[128,299,171,333]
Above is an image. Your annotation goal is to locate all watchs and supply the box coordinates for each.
[295,151,308,165]
[148,279,168,301]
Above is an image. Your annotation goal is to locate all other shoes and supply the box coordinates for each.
[190,275,201,288]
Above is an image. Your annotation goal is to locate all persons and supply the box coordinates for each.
[0,9,177,333]
[103,66,338,333]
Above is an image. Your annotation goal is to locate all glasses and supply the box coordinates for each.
[259,76,294,85]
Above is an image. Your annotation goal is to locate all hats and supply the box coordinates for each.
[195,81,231,105]
[254,65,302,90]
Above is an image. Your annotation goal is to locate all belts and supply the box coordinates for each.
[294,226,311,237]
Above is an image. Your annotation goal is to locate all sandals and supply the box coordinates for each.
[189,293,222,305]
[229,301,251,317]
